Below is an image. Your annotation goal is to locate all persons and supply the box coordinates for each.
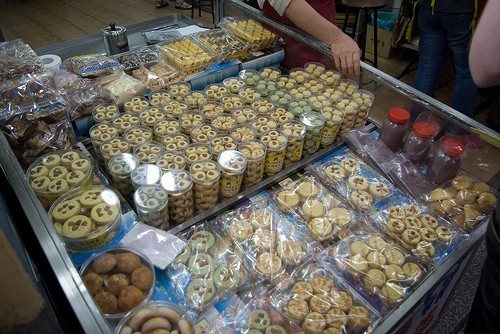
[464,0,500,334]
[401,0,487,148]
[257,0,361,77]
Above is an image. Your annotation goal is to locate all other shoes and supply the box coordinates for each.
[155,0,169,8]
[175,1,192,10]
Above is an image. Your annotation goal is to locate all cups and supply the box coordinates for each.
[89,63,375,231]
[102,21,129,56]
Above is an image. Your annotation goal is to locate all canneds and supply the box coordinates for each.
[431,137,465,184]
[378,107,410,152]
[404,121,436,164]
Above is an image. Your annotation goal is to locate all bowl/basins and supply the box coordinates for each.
[113,300,196,334]
[79,246,156,319]
[47,185,123,253]
[25,148,94,209]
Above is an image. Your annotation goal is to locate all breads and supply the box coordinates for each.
[0,18,497,334]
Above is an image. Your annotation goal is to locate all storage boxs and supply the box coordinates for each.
[187,45,286,91]
[366,23,397,59]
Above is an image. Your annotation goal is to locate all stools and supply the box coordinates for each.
[341,0,387,86]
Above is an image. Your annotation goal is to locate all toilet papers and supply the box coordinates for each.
[38,54,63,70]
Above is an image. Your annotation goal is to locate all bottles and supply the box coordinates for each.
[403,121,439,162]
[425,137,466,185]
[381,106,412,153]
